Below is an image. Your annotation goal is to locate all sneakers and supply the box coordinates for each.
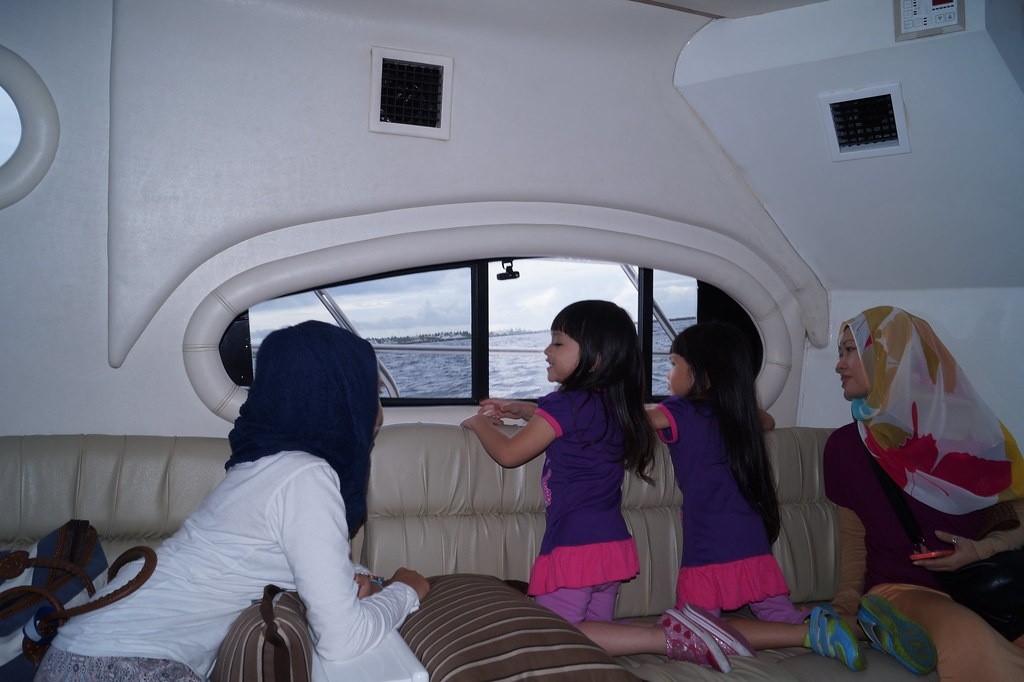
[681,601,757,658]
[803,603,867,672]
[857,594,938,675]
[656,608,732,674]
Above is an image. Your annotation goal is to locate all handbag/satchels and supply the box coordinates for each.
[0,518,157,682]
[944,545,1024,642]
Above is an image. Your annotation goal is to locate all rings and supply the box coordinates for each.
[952,535,959,544]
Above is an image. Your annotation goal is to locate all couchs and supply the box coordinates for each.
[0,421,941,682]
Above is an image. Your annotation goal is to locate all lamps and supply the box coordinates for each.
[497,261,520,280]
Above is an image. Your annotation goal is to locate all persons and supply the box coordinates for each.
[36,321,428,682]
[646,325,938,675]
[823,307,1024,682]
[461,300,756,673]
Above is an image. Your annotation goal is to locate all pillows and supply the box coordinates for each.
[397,573,649,682]
[208,592,314,682]
